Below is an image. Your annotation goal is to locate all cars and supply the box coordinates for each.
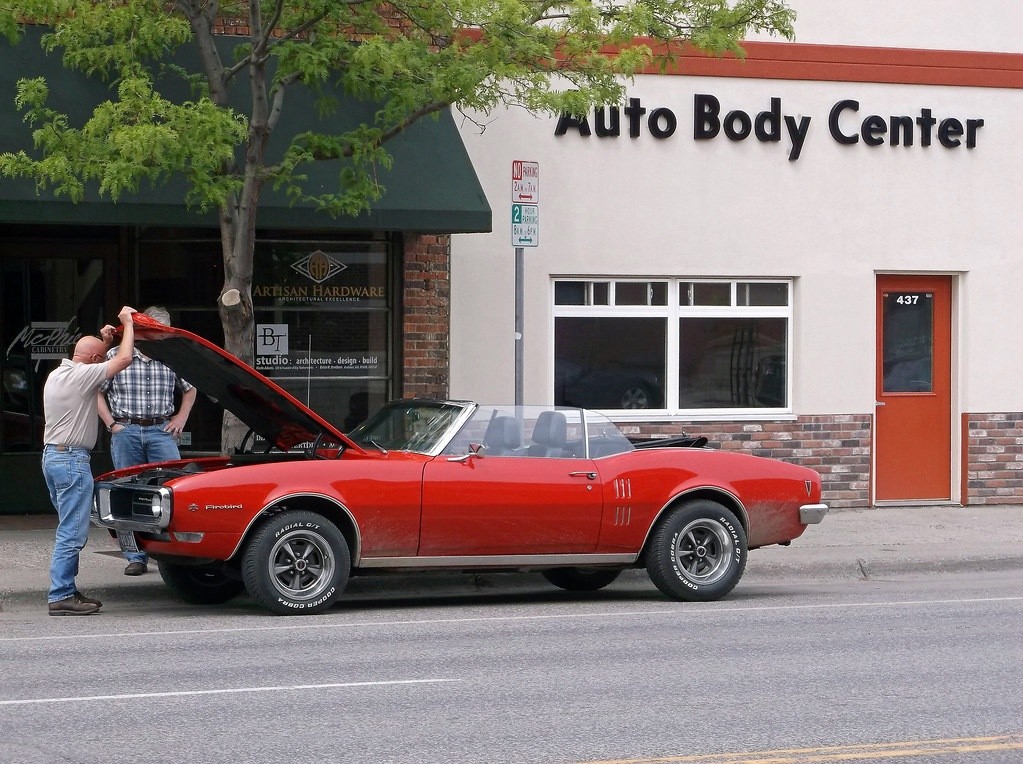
[89,315,830,612]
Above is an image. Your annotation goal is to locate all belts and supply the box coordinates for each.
[112,416,169,427]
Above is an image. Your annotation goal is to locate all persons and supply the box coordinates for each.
[97,305,198,576]
[42,305,137,616]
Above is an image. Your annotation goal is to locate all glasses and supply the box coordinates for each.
[90,353,108,362]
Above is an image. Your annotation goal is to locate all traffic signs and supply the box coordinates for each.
[512,203,539,248]
[511,160,540,204]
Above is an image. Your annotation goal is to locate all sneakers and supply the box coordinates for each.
[48,596,100,616]
[124,563,147,576]
[74,590,103,607]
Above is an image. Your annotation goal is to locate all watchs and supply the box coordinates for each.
[107,422,116,432]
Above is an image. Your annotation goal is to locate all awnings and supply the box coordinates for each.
[0,22,493,234]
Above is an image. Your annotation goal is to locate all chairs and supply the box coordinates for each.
[528,411,567,457]
[484,417,521,457]
